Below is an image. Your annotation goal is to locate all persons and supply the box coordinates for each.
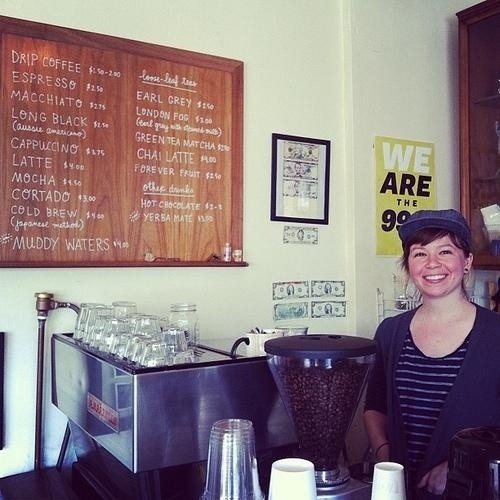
[363,209,500,500]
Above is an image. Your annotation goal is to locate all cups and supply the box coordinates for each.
[72,302,200,367]
[232,250,242,262]
[200,418,406,500]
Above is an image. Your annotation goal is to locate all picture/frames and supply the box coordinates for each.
[270,133,331,226]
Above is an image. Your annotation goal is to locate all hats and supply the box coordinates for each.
[398,209,472,249]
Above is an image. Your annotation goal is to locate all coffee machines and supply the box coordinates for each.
[264,334,379,499]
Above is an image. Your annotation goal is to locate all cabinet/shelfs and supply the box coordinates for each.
[455,0,500,271]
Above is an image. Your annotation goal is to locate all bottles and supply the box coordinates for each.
[223,243,231,262]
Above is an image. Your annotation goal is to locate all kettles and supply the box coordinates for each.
[442,427,499,500]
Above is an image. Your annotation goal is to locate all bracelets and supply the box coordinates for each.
[375,442,390,457]
